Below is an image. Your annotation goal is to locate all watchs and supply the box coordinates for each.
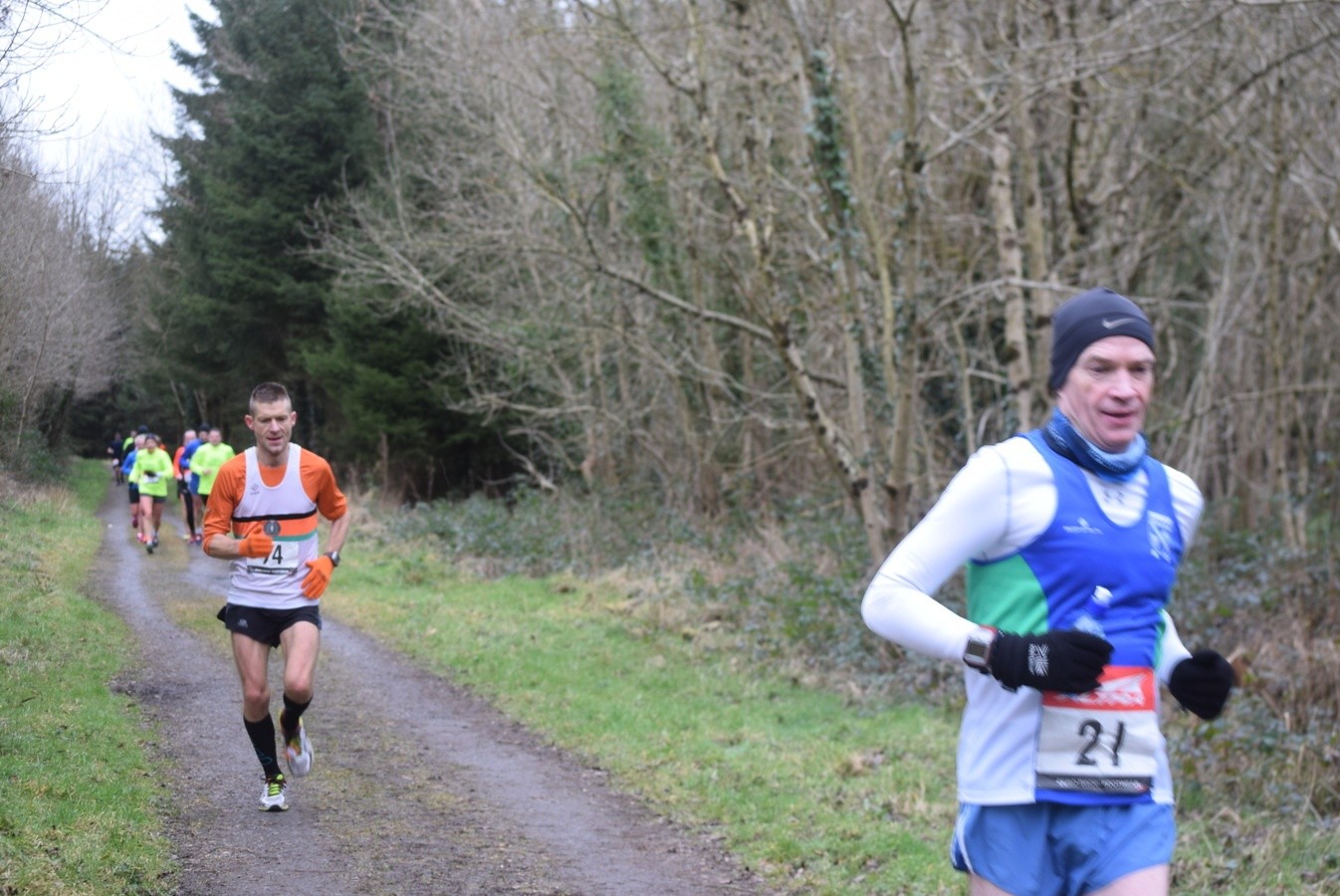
[322,550,341,567]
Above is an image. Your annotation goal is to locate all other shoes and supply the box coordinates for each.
[182,534,190,540]
[189,536,195,542]
[132,516,138,527]
[143,536,147,541]
[195,534,202,543]
[146,541,153,553]
[153,537,159,546]
[137,532,144,542]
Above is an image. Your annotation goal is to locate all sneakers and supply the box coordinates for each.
[258,774,288,811]
[277,707,314,778]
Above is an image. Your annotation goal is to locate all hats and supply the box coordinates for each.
[137,425,148,435]
[199,424,210,434]
[145,434,161,446]
[1048,287,1155,390]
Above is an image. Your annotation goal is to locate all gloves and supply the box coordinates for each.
[1170,650,1235,720]
[303,555,334,600]
[240,526,273,558]
[989,631,1114,694]
[144,470,155,478]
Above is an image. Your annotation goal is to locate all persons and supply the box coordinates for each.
[188,426,236,537]
[130,433,174,554]
[202,381,351,812]
[858,289,1235,896]
[107,420,210,544]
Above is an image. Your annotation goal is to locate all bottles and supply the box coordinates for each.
[1071,585,1113,641]
